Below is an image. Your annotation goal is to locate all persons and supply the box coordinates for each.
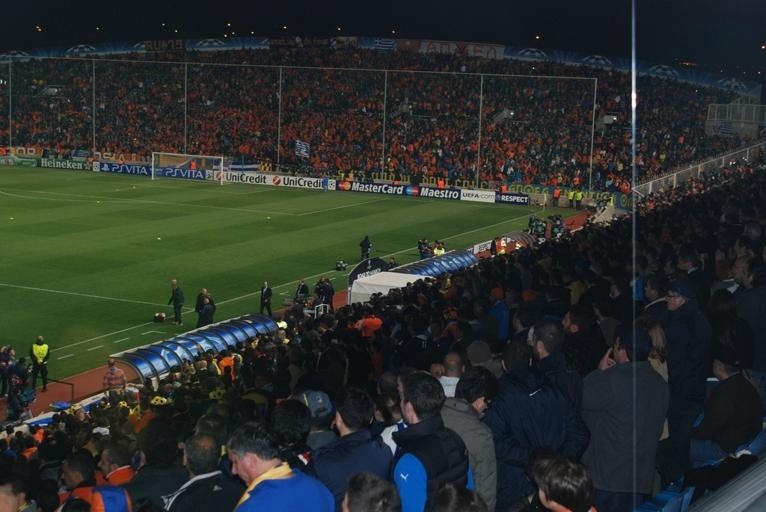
[1,45,766,193]
[2,148,764,512]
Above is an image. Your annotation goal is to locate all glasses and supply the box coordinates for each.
[663,292,678,300]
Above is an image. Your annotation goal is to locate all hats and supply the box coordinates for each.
[466,340,491,363]
[673,279,698,298]
[297,389,332,418]
[707,343,740,366]
